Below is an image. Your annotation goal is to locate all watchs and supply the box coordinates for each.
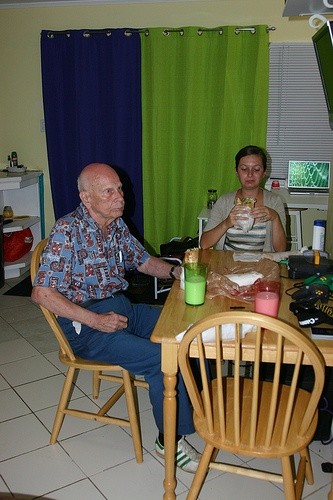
[170,265,178,279]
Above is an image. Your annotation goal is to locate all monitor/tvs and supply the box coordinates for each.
[312,20,333,123]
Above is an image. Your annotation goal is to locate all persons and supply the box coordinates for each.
[199,145,287,251]
[31,163,212,476]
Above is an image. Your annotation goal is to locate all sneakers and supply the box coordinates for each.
[155,434,203,473]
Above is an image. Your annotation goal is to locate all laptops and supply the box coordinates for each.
[287,160,330,196]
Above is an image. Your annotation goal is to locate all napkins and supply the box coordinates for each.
[176,322,257,343]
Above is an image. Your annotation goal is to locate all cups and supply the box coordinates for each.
[254,292,279,319]
[184,261,209,306]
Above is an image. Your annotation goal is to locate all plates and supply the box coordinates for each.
[8,168,26,172]
[26,169,42,172]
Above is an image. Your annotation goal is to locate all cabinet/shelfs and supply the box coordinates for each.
[0,171,45,279]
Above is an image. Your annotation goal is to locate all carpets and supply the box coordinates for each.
[2,274,172,305]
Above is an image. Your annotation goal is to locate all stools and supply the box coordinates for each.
[284,208,308,251]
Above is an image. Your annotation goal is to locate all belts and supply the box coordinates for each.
[79,291,122,308]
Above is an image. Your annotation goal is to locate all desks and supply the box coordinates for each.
[150,249,333,500]
[198,208,212,249]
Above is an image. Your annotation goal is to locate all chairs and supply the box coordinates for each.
[176,308,326,500]
[30,239,150,464]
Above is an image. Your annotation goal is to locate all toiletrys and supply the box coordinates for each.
[312,219,327,251]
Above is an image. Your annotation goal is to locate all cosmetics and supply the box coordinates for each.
[313,220,326,250]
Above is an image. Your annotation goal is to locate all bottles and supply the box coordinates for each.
[207,189,218,209]
[3,205,13,220]
[271,180,280,190]
[279,259,288,278]
[8,152,18,168]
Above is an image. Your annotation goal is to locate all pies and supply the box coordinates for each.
[185,248,200,263]
[234,197,257,209]
